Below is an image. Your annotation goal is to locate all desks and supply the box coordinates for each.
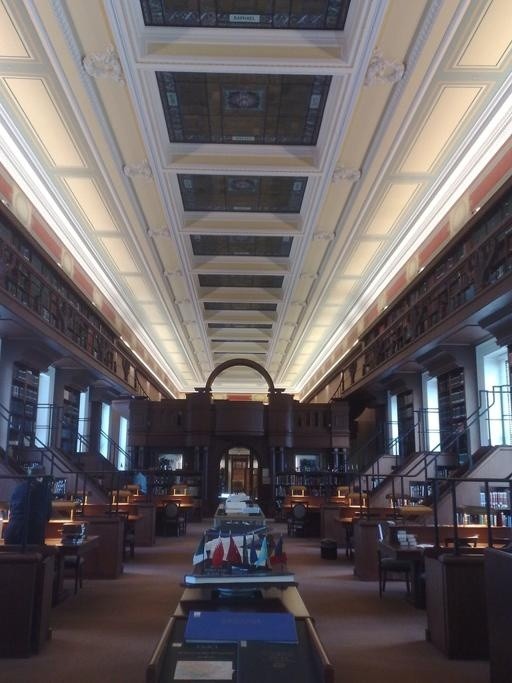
[380,538,506,610]
[45,535,101,594]
[128,514,144,522]
[156,504,194,511]
[284,504,320,513]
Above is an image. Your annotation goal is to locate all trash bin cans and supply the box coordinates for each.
[320,539,337,560]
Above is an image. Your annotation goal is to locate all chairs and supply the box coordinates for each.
[287,500,309,537]
[375,522,413,599]
[161,498,185,536]
[107,511,135,560]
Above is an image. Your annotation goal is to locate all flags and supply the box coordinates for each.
[192,533,288,568]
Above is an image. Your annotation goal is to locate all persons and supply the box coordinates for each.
[3,465,55,544]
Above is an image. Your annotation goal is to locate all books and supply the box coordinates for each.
[456,491,512,527]
[397,530,416,546]
[61,523,86,544]
